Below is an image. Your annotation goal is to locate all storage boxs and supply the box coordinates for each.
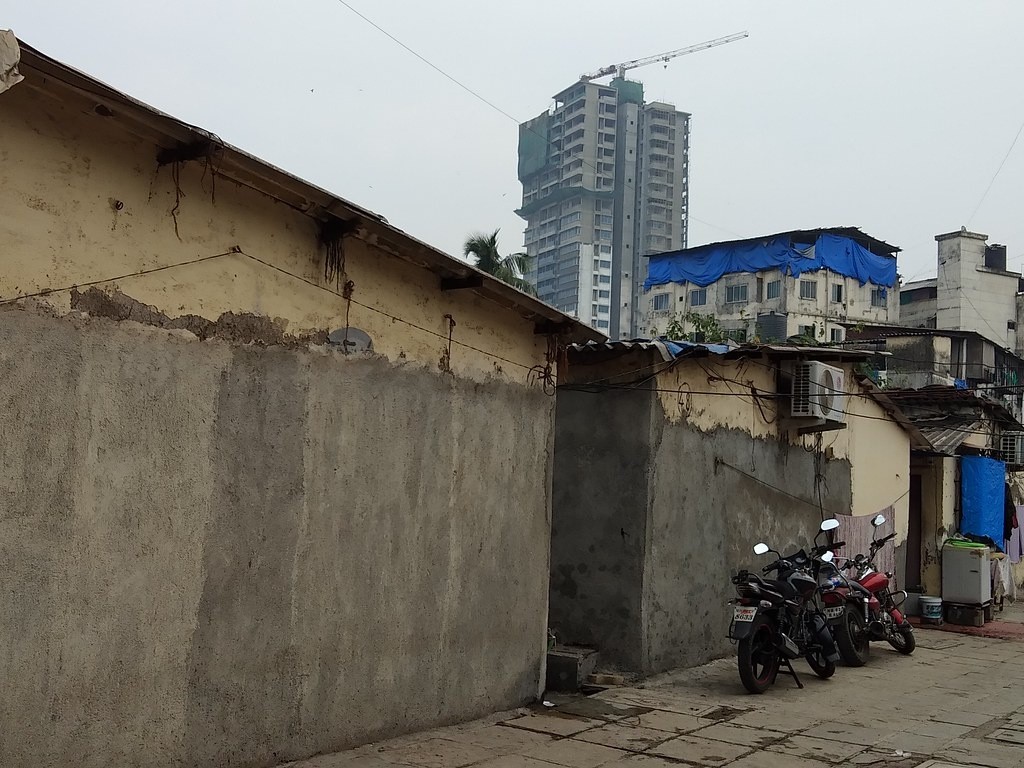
[942,545,991,604]
[919,596,941,619]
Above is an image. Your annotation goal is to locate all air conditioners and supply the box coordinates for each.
[791,360,845,423]
[1000,430,1024,465]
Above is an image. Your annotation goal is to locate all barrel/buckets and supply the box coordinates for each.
[920,596,943,620]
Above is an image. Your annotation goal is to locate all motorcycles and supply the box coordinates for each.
[723,510,916,696]
